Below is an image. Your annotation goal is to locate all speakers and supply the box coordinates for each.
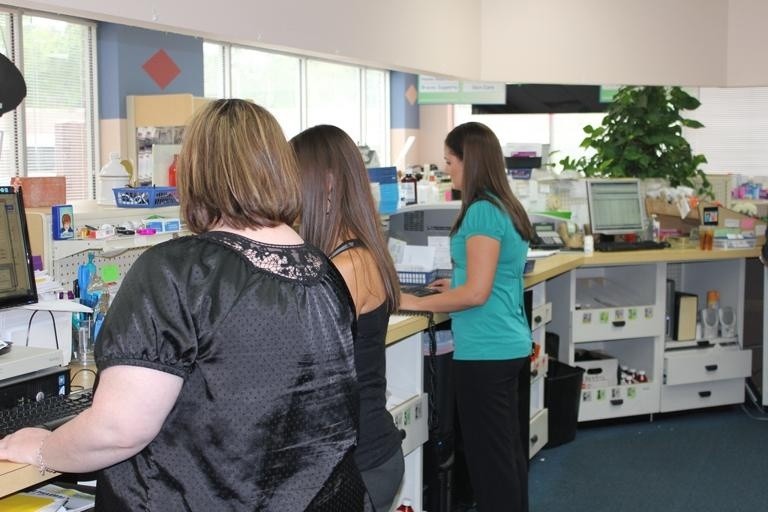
[719,306,737,338]
[701,308,719,338]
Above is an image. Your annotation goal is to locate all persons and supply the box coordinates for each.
[285,125,404,511]
[1,97,368,512]
[395,121,542,510]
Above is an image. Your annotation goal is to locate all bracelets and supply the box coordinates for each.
[35,431,57,474]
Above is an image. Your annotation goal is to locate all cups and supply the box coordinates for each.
[624,233,641,244]
[698,228,714,249]
[76,319,98,365]
[582,235,594,255]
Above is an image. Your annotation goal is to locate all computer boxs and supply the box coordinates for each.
[0,366,71,409]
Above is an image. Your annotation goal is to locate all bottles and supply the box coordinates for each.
[706,290,720,311]
[168,153,180,187]
[621,365,647,381]
[95,153,131,207]
[400,173,438,205]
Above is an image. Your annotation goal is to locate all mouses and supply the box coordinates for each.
[660,242,671,247]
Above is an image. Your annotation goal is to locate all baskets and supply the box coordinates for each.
[113,187,177,208]
[396,267,437,285]
[437,269,452,279]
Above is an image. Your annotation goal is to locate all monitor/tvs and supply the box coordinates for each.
[0,185,39,310]
[586,178,645,243]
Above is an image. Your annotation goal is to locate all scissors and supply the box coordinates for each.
[76,264,90,304]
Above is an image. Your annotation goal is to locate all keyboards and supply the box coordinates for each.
[400,286,440,298]
[0,387,94,440]
[593,241,660,252]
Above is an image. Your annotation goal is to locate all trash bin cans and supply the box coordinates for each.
[544,359,586,449]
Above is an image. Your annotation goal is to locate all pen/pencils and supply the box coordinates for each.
[59,279,99,320]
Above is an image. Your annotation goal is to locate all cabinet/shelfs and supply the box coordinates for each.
[123,95,207,198]
[26,198,193,318]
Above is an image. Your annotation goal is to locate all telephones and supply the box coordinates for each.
[529,222,566,250]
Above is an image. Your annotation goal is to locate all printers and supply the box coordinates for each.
[390,204,462,280]
